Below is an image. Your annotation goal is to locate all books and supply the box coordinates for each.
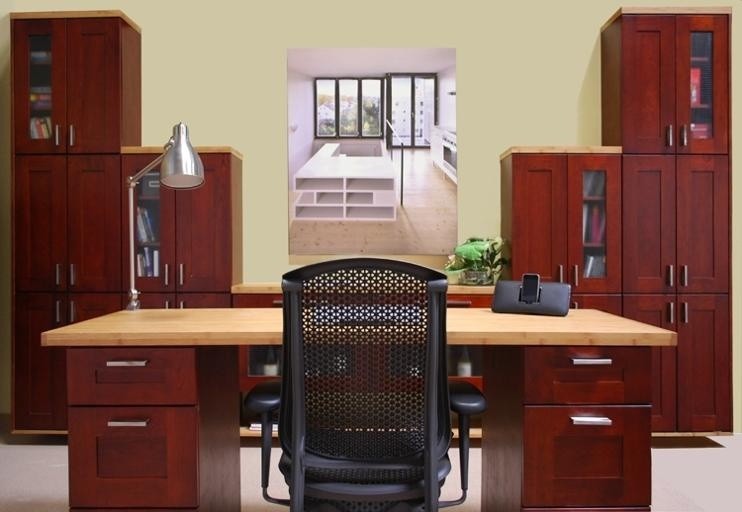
[29,52,53,140]
[582,170,606,277]
[249,415,278,431]
[137,206,160,277]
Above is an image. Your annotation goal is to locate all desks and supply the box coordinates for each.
[42,307,679,511]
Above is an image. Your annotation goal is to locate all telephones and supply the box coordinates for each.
[491,273,571,316]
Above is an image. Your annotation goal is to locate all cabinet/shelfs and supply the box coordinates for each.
[624,295,732,436]
[231,293,497,448]
[500,144,623,316]
[60,346,242,510]
[623,152,730,295]
[9,18,143,155]
[292,142,396,220]
[12,157,130,432]
[598,6,732,155]
[121,144,244,312]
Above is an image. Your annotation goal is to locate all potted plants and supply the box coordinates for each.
[443,237,509,287]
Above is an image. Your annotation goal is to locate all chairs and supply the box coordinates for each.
[242,256,487,512]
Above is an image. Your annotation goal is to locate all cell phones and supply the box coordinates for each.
[521,273,541,302]
